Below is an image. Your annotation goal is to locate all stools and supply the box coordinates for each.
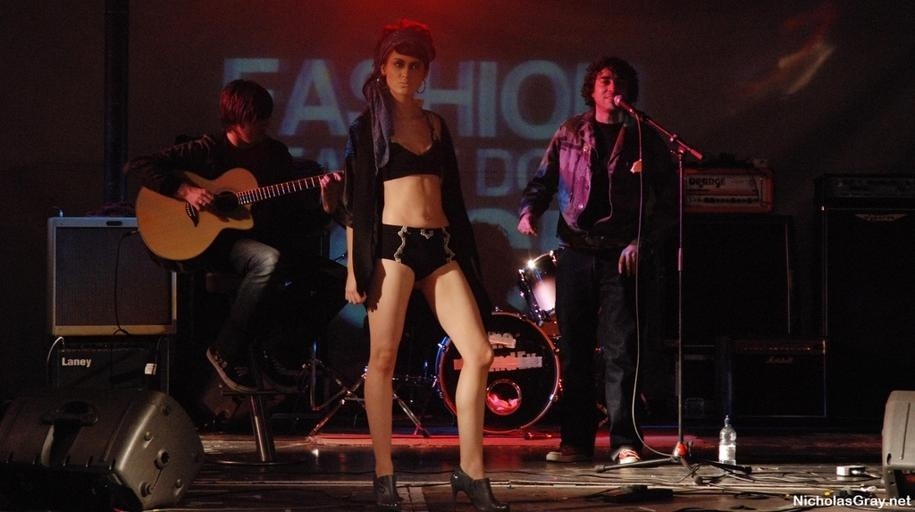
[205,271,309,466]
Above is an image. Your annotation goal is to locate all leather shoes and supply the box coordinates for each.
[373,471,401,508]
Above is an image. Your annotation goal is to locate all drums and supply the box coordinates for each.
[517,247,563,339]
[430,306,564,434]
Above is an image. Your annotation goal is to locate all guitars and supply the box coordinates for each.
[136,168,345,261]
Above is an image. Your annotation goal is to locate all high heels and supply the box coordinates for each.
[450,466,509,511]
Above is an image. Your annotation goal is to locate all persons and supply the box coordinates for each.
[513,53,671,462]
[341,17,514,512]
[137,77,344,395]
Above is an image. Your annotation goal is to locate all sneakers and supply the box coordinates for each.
[546,446,577,462]
[619,449,639,464]
[206,347,258,394]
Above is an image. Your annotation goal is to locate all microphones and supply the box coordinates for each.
[614,95,639,121]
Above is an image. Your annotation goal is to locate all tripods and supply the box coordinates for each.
[595,146,751,485]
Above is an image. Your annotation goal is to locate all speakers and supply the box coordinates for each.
[44,337,169,399]
[681,214,798,346]
[0,389,204,512]
[47,217,177,336]
[717,341,828,420]
[681,348,728,432]
[816,207,915,419]
[881,390,915,502]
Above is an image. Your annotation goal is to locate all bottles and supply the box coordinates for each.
[718,414,738,465]
